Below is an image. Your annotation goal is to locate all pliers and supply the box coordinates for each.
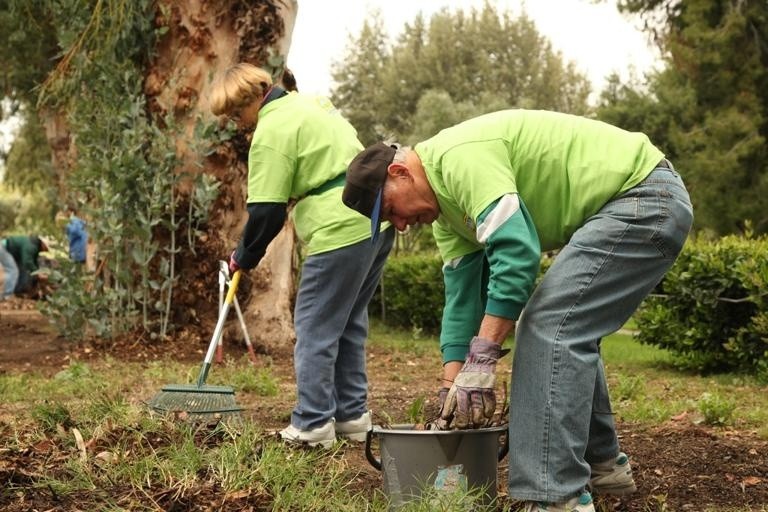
[216,259,258,363]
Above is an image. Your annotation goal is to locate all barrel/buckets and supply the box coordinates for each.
[365,421,510,510]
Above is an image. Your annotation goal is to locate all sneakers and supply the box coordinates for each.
[334,408,376,444]
[269,417,337,450]
[523,489,596,512]
[584,452,638,495]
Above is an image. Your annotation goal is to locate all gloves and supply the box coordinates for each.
[429,335,513,432]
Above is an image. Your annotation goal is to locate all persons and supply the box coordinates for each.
[342,108,694,512]
[1,233,50,302]
[209,63,395,451]
[61,207,88,268]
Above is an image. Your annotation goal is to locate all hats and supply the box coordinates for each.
[342,141,399,246]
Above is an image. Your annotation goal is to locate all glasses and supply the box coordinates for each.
[228,105,245,122]
